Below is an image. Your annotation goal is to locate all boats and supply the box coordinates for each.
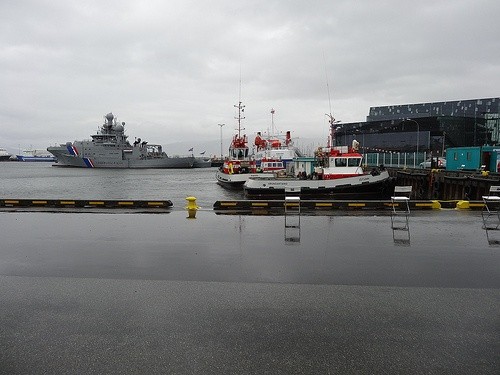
[243,140,397,201]
[215,100,298,191]
[9,150,58,161]
[0,146,12,160]
[46,112,195,168]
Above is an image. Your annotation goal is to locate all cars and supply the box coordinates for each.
[419,157,448,170]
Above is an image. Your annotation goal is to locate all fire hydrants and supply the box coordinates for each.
[185,196,201,219]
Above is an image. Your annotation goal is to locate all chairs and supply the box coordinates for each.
[284,187,300,214]
[391,186,412,215]
[482,186,500,217]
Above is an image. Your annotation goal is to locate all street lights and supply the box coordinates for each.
[218,123,225,162]
[406,118,420,164]
